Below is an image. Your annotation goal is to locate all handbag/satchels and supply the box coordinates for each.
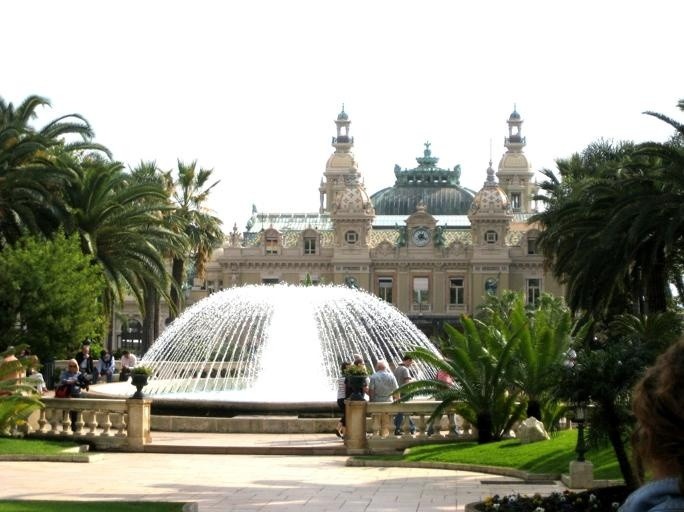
[55,384,70,398]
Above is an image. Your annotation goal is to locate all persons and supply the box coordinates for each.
[0,337,137,437]
[335,352,457,439]
[620,329,684,511]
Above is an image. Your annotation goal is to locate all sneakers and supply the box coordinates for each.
[335,428,342,437]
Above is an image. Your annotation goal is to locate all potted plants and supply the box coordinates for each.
[132,367,152,398]
[389,307,576,444]
[343,365,368,400]
